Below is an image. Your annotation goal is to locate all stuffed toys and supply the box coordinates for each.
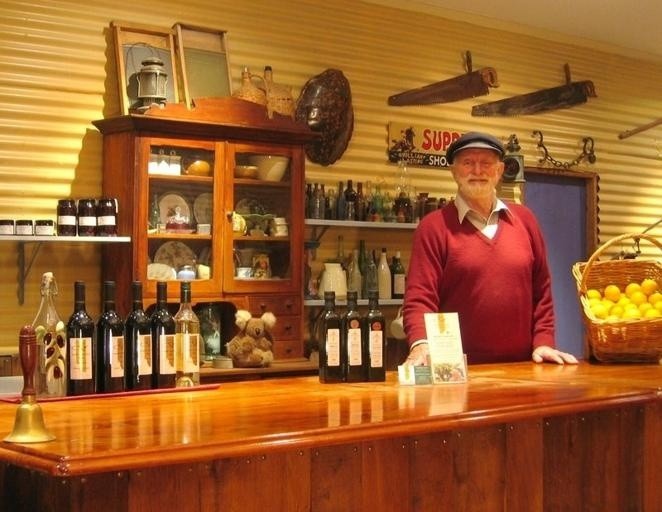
[227,307,277,368]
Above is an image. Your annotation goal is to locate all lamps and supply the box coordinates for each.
[126,42,169,113]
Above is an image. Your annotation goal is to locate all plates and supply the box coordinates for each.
[147,191,268,280]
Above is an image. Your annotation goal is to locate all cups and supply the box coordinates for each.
[148,149,181,176]
[0,354,12,376]
[391,305,407,339]
[12,357,23,376]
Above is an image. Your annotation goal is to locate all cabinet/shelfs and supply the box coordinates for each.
[90,114,314,365]
[304,215,418,310]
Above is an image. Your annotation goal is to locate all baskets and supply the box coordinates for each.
[572,233,662,362]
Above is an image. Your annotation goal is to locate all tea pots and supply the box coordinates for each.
[181,155,212,176]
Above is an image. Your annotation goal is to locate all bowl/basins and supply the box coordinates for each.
[235,165,259,178]
[249,156,290,182]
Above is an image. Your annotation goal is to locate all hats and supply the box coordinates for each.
[447,131,505,164]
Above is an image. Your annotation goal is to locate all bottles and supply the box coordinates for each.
[305,180,448,224]
[35,273,221,399]
[319,291,385,383]
[0,198,118,237]
[319,235,407,299]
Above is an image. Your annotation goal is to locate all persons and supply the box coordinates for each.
[404,131,579,367]
